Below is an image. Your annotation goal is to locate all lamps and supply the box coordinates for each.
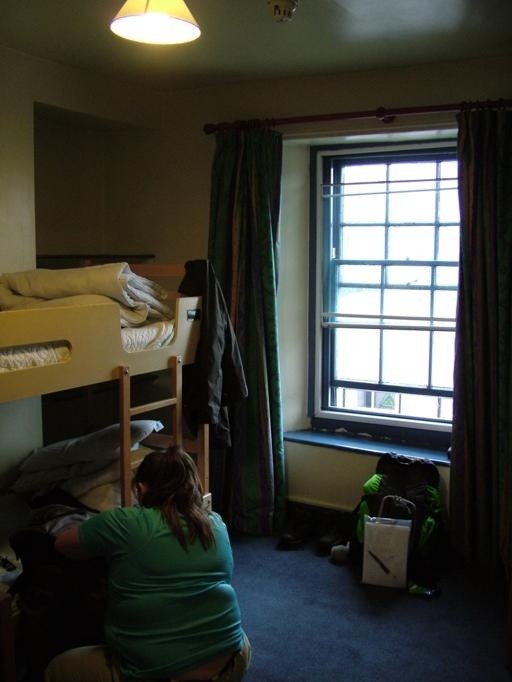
[108,0,203,46]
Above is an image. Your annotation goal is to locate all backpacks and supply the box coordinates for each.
[353,453,448,599]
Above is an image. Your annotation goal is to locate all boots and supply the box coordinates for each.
[316,510,353,554]
[279,505,317,549]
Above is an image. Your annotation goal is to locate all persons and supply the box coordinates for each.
[43,443,252,682]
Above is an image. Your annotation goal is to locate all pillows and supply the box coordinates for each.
[29,416,166,468]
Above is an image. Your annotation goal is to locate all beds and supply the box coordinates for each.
[1,263,212,671]
